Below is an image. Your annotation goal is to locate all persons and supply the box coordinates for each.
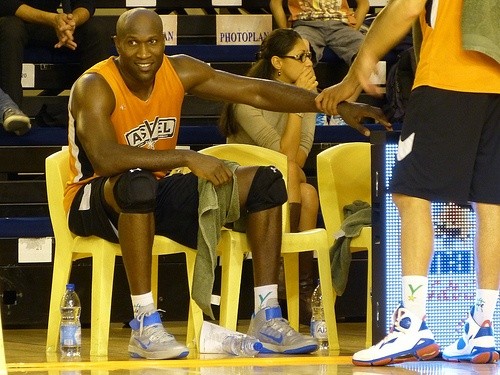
[270,0,370,125]
[314,0,500,367]
[219,28,318,326]
[66,8,391,361]
[0,0,108,112]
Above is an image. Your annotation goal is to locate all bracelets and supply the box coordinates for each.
[296,113,305,117]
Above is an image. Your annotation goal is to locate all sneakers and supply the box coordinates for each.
[351,307,440,366]
[248,306,320,354]
[127,308,190,360]
[442,306,500,364]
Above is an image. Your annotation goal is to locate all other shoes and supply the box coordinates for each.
[3,109,32,135]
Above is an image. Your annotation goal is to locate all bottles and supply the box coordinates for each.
[311,279,329,350]
[221,334,264,358]
[59,284,80,357]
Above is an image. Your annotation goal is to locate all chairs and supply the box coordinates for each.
[0,0,372,361]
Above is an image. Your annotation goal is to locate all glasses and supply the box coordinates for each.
[271,51,313,62]
